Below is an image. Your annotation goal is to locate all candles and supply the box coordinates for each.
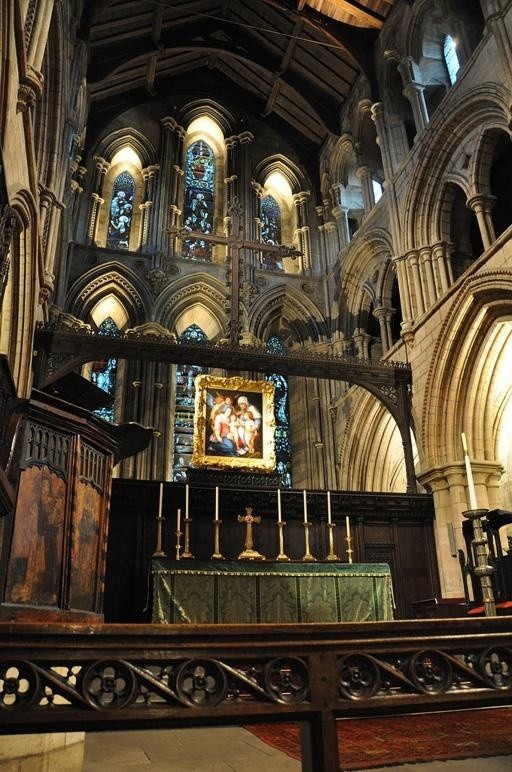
[185,484,190,520]
[346,515,351,538]
[326,490,332,524]
[277,488,282,522]
[158,482,164,518]
[461,432,478,509]
[177,508,182,532]
[215,486,220,521]
[303,489,307,523]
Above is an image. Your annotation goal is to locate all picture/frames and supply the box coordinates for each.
[192,373,279,473]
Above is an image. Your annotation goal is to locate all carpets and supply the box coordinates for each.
[238,704,512,772]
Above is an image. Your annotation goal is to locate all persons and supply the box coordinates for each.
[207,396,261,457]
[34,475,58,604]
